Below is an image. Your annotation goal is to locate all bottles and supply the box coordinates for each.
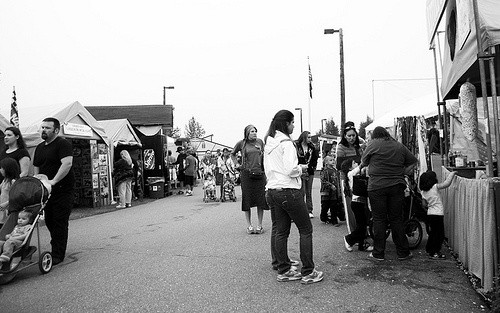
[448,150,453,167]
[449,150,467,167]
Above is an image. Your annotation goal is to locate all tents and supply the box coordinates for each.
[97,119,144,205]
[0,100,116,205]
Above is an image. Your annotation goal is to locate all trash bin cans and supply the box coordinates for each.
[147,177,165,198]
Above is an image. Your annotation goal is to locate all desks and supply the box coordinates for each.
[447,166,486,179]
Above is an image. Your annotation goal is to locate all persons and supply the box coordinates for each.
[342,158,374,251]
[132,158,139,200]
[0,209,36,263]
[33,118,75,265]
[362,126,419,260]
[0,127,32,178]
[418,170,459,258]
[218,147,234,200]
[114,150,134,208]
[318,122,367,226]
[296,131,319,218]
[232,125,264,234]
[205,176,215,198]
[0,158,20,228]
[201,150,217,201]
[165,146,200,196]
[223,180,234,194]
[264,110,323,285]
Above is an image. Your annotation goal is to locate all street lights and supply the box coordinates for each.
[163,86,174,105]
[323,28,346,139]
[295,107,302,134]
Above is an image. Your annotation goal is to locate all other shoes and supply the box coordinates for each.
[344,236,353,251]
[116,204,132,209]
[185,190,193,196]
[309,212,314,218]
[398,251,413,260]
[431,252,445,259]
[369,253,385,261]
[289,258,300,270]
[52,257,64,265]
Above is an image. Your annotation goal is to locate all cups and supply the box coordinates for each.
[299,164,308,171]
[469,161,475,168]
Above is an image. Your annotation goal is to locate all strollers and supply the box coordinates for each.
[368,173,423,251]
[0,176,53,286]
[220,168,237,202]
[202,171,220,203]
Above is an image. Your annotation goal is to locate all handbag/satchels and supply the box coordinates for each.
[352,174,369,197]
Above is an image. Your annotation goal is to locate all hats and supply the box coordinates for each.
[176,146,183,152]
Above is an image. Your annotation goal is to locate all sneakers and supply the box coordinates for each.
[301,269,324,284]
[247,225,254,233]
[276,270,301,282]
[255,224,264,234]
